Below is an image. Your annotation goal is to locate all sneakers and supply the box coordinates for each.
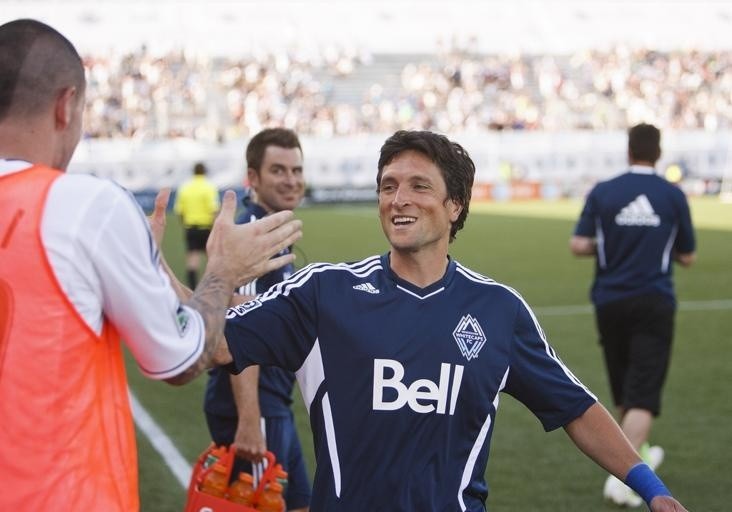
[640,443,663,472]
[605,475,643,507]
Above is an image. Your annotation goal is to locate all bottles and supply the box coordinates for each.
[226,472,254,507]
[265,464,287,493]
[200,465,226,498]
[254,483,285,512]
[202,446,226,471]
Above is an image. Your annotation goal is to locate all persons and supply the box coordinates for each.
[75,44,369,135]
[172,162,221,291]
[145,129,690,511]
[200,129,312,509]
[361,52,732,130]
[569,122,701,507]
[1,18,304,511]
